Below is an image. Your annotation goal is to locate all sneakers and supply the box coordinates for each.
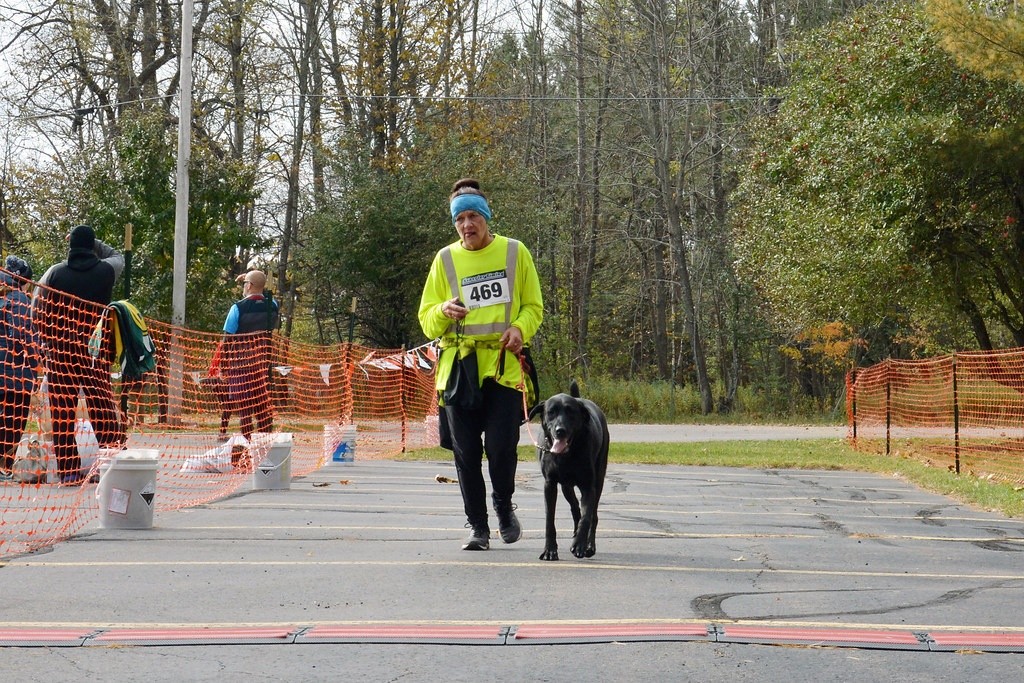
[492,499,522,544]
[462,515,490,549]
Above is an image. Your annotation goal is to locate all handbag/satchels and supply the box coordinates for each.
[74,418,100,476]
[12,430,46,483]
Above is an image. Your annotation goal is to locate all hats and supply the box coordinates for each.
[5,255,33,288]
[69,226,95,250]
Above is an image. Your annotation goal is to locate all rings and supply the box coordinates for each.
[514,343,518,347]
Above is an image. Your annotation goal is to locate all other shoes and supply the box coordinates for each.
[218,434,230,442]
[61,474,82,487]
[0,469,12,478]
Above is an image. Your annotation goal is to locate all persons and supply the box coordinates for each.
[418,178,543,550]
[30,225,129,485]
[0,255,44,481]
[208,272,282,442]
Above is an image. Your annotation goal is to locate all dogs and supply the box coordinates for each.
[527,379,611,561]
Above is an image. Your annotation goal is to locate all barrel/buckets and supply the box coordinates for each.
[324,424,357,467]
[98,448,160,531]
[424,415,440,447]
[250,432,292,491]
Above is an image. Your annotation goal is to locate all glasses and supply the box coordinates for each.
[242,280,253,286]
